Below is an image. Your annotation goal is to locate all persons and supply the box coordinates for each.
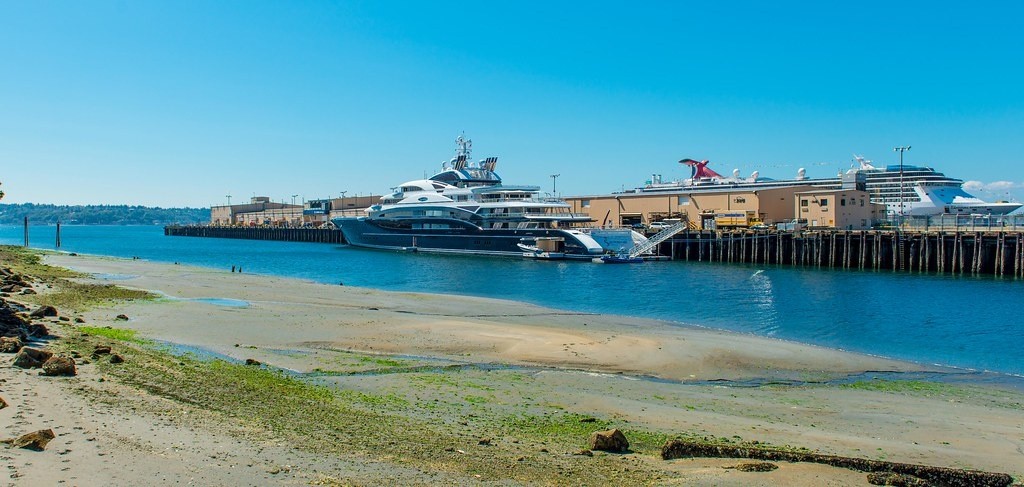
[232,265,242,272]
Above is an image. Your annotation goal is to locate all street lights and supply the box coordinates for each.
[893,146,910,219]
[551,172,562,197]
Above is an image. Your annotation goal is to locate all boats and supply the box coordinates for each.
[331,130,672,263]
[826,164,1023,225]
